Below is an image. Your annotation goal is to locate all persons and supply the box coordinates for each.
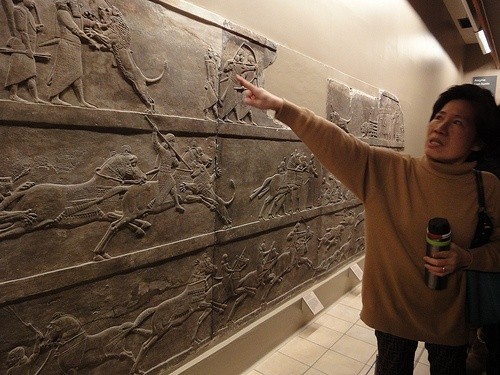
[465,104,500,375]
[4,0,48,104]
[236,74,500,375]
[287,223,307,264]
[147,125,185,213]
[45,0,100,109]
[217,48,258,124]
[204,43,225,124]
[278,150,318,176]
[256,241,275,284]
[240,54,258,126]
[221,254,234,277]
[6,331,43,375]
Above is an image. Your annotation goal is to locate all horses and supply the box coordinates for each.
[250,163,320,222]
[0,150,151,241]
[91,146,215,263]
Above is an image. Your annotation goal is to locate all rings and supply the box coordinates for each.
[443,266,448,272]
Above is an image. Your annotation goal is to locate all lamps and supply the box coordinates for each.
[472,27,492,55]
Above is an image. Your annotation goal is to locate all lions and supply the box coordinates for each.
[178,160,238,230]
[84,5,165,113]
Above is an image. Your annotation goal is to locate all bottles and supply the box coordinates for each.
[423,217,451,291]
